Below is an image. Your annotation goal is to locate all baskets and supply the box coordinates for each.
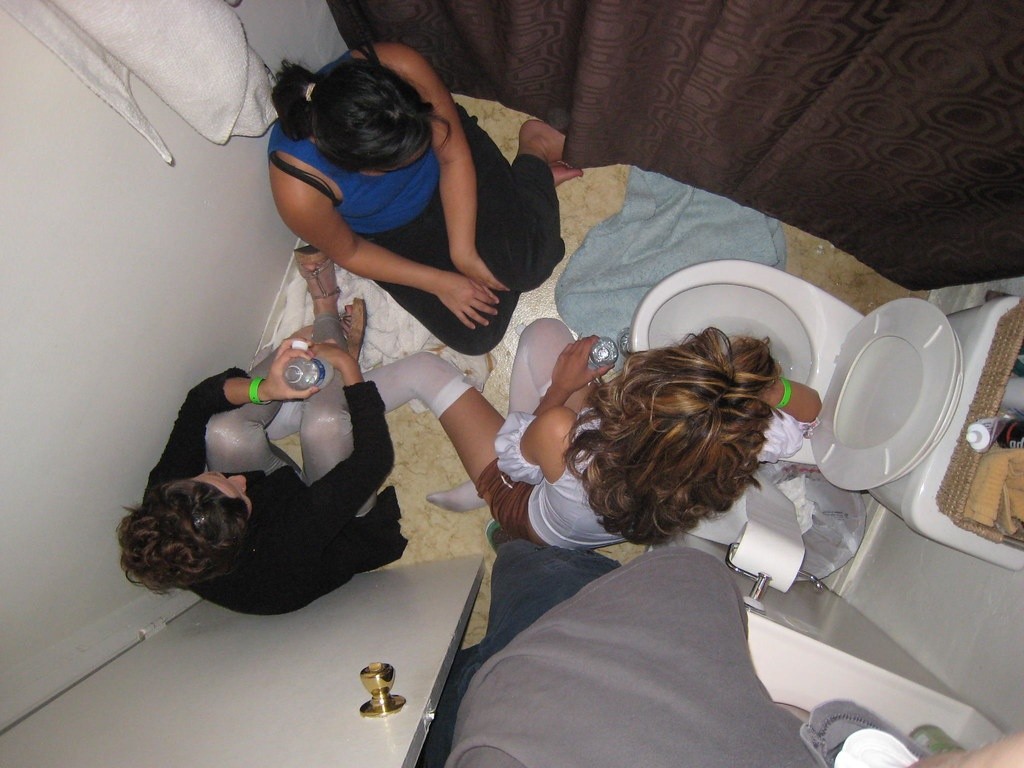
[934,301,1024,552]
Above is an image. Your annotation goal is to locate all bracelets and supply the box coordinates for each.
[773,377,791,410]
[249,376,270,405]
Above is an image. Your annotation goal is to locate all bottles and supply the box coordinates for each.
[281,357,334,390]
[617,326,631,354]
[909,723,967,760]
[588,336,619,367]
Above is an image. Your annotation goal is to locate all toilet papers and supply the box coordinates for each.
[728,469,806,595]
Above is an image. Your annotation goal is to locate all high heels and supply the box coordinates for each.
[291,245,342,303]
[336,295,367,364]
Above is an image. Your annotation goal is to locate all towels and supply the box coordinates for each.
[965,447,1024,538]
[551,164,789,375]
[257,241,498,417]
[1,0,282,168]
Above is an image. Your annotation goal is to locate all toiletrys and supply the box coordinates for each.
[964,406,1024,454]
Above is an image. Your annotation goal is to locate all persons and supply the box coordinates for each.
[116,244,408,617]
[419,521,1024,768]
[266,317,824,553]
[267,41,585,357]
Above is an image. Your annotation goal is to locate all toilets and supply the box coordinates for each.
[627,255,1024,574]
